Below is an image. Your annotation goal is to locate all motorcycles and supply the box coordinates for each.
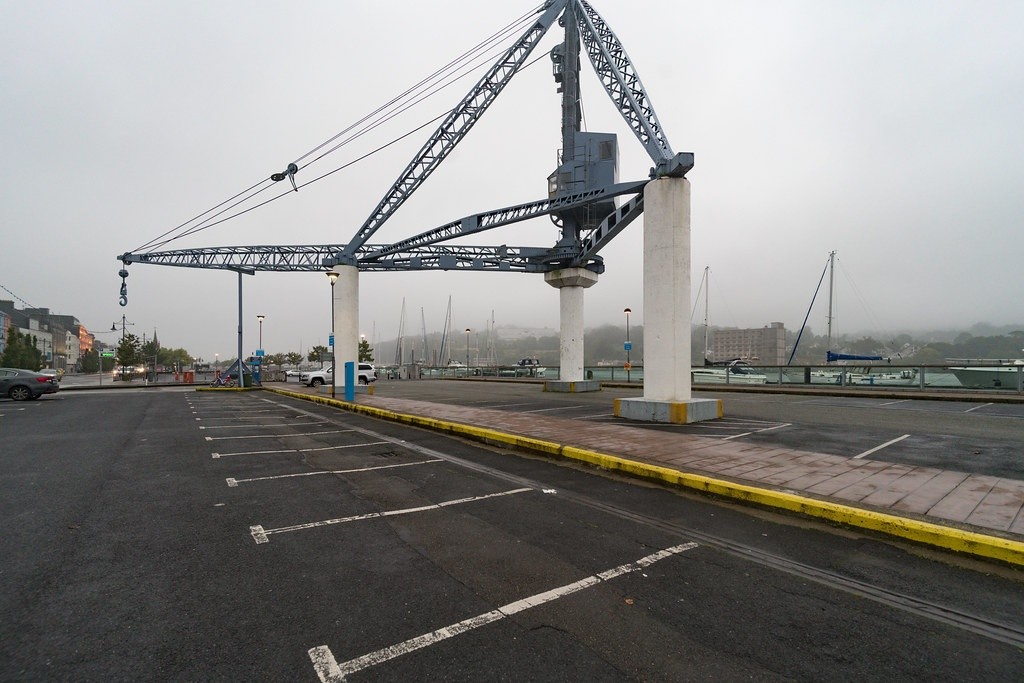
[210,373,234,388]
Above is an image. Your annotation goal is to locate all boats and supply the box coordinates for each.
[948,367,1024,389]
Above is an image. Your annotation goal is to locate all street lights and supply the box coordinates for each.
[466,328,471,377]
[360,333,365,346]
[256,314,265,349]
[623,308,635,382]
[110,314,135,373]
[216,353,219,372]
[325,270,341,398]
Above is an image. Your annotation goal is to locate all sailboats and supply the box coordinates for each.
[368,292,547,377]
[690,266,770,385]
[782,250,932,386]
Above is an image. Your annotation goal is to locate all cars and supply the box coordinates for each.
[113,365,135,375]
[0,367,60,400]
[35,368,62,383]
[301,360,378,388]
[286,369,303,377]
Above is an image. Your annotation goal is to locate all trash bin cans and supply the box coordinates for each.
[147,370,155,383]
[182,370,195,382]
[112,374,122,382]
[238,373,253,387]
